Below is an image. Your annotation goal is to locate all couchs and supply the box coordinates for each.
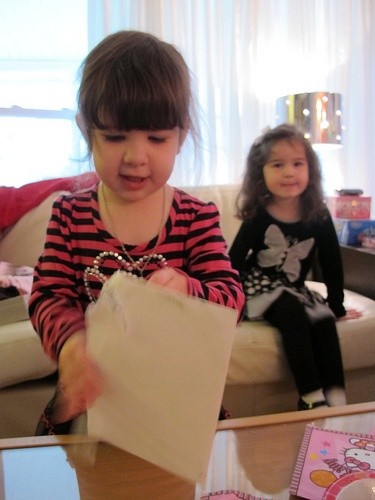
[0,184,375,439]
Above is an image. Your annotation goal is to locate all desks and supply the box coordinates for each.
[338,243,375,301]
[1,402,375,500]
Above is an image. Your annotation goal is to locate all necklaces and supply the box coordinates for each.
[102,181,166,278]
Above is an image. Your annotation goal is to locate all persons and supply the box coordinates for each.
[228,123,365,411]
[28,30,246,436]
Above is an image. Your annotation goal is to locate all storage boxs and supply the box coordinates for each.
[336,197,372,219]
[0,294,29,325]
[339,220,375,243]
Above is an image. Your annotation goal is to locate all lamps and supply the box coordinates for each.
[288,91,342,198]
